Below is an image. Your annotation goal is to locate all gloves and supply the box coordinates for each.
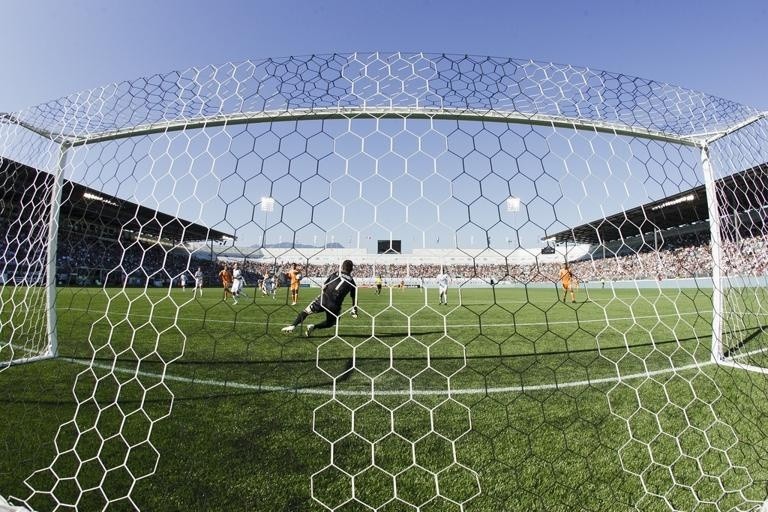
[351,308,357,319]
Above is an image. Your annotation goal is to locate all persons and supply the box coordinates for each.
[218,264,232,302]
[601,278,606,288]
[375,273,383,296]
[179,274,189,292]
[558,263,578,304]
[285,262,301,305]
[257,278,268,296]
[281,257,361,339]
[230,262,249,306]
[397,280,408,289]
[193,266,205,297]
[262,274,271,296]
[271,273,277,301]
[432,266,452,307]
[418,277,425,294]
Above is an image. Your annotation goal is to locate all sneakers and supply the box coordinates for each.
[305,324,314,336]
[281,325,295,335]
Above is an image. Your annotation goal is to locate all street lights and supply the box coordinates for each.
[506,197,520,248]
[260,196,274,248]
